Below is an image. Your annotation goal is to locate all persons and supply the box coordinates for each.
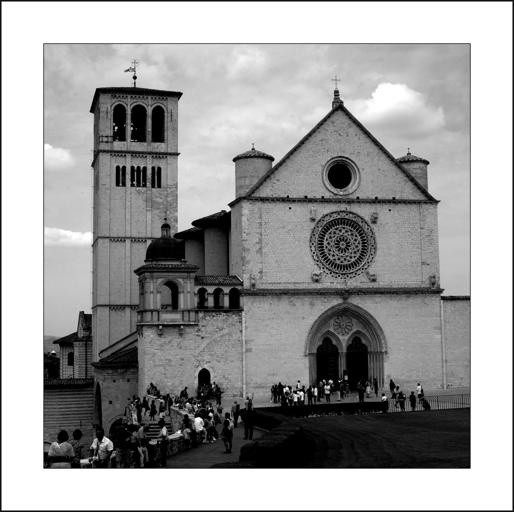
[409,391,416,411]
[416,383,423,404]
[270,377,379,408]
[381,393,388,402]
[420,398,430,411]
[419,389,424,403]
[398,391,406,412]
[394,385,400,409]
[47,381,256,469]
[388,378,397,400]
